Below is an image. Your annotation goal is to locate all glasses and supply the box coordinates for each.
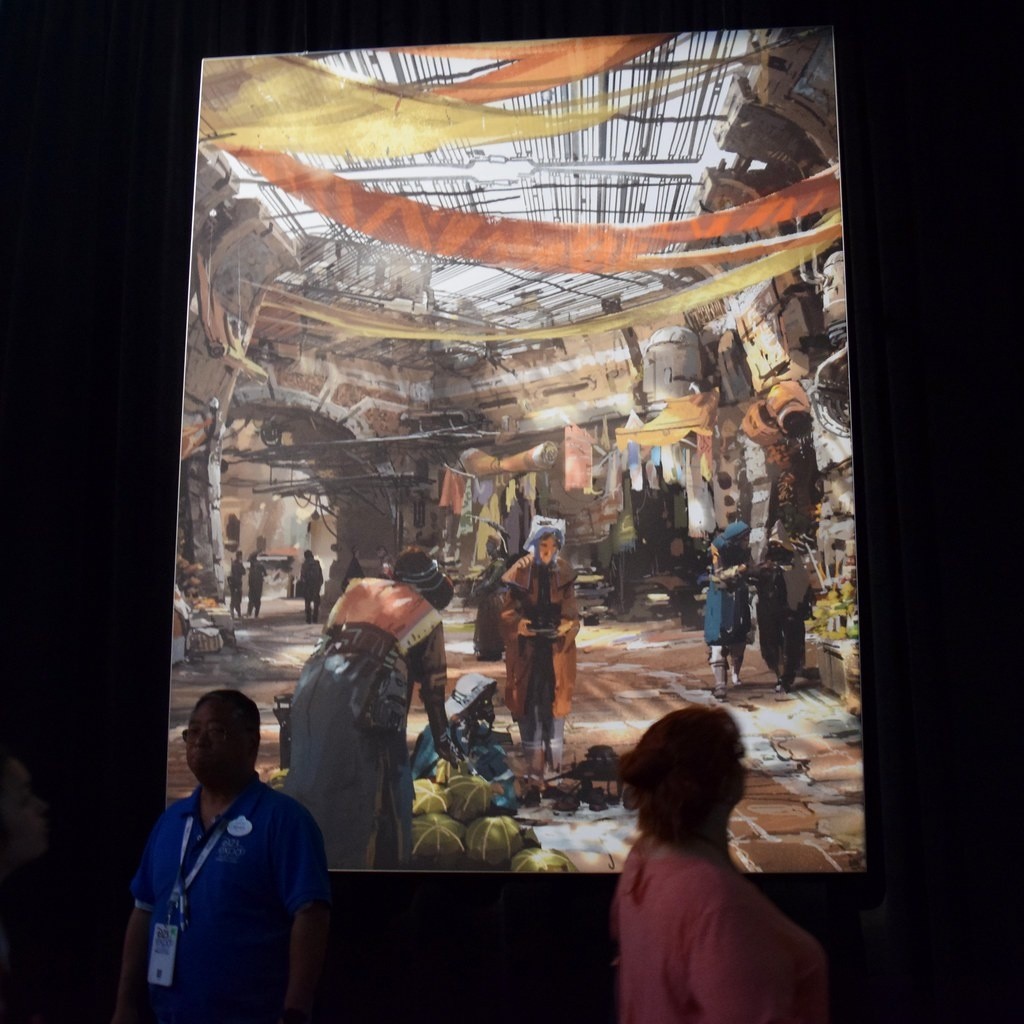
[183,726,243,744]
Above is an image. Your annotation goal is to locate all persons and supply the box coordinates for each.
[611,705,830,1024]
[0,744,49,1024]
[111,690,332,1024]
